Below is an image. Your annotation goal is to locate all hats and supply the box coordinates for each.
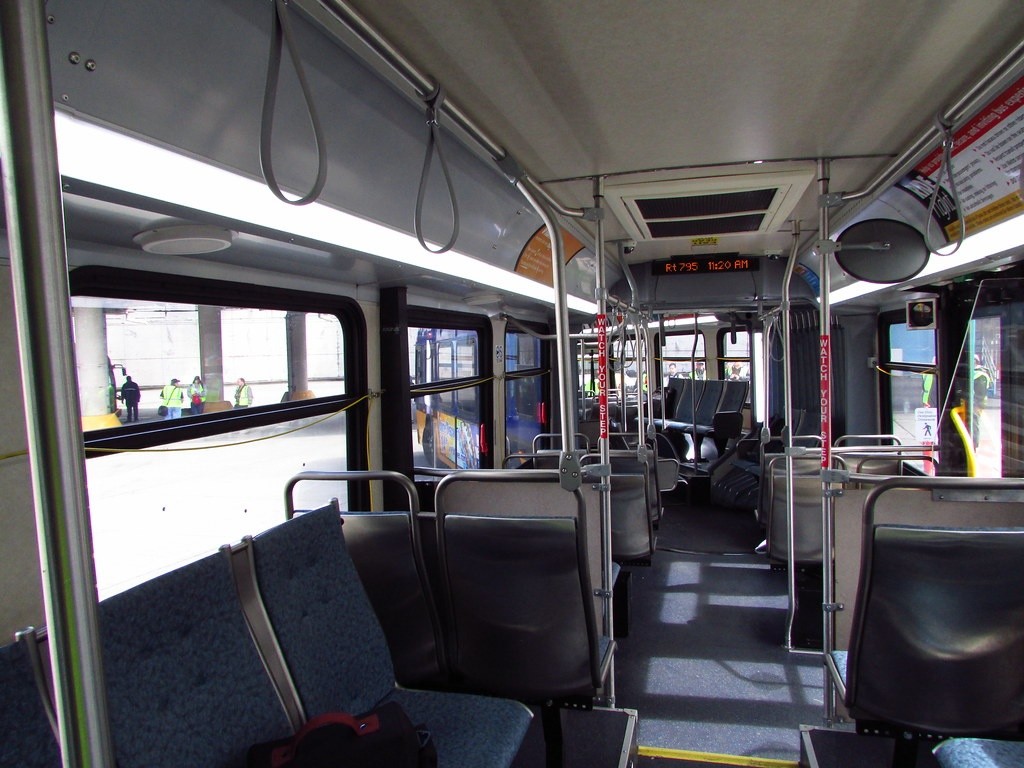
[733,363,743,369]
[171,379,180,384]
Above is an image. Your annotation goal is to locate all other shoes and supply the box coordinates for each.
[125,420,132,423]
[135,419,141,421]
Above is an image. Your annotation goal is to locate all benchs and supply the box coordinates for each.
[755,435,1024,768]
[11,378,749,767]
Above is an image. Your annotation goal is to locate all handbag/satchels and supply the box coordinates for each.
[248,701,438,768]
[158,406,168,416]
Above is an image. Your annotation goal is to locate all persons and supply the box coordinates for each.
[724,361,746,382]
[922,358,937,408]
[187,376,207,415]
[583,370,600,395]
[234,377,253,409]
[121,376,141,423]
[159,379,184,420]
[688,361,707,380]
[665,363,683,387]
[973,353,990,454]
